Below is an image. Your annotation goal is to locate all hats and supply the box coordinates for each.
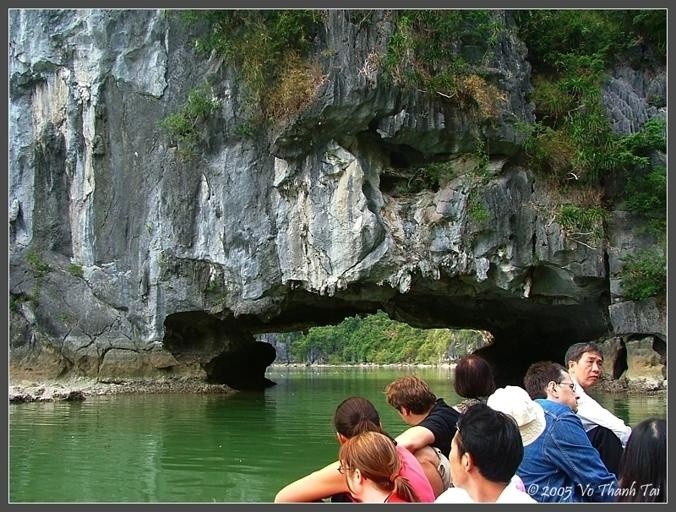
[487,385,546,447]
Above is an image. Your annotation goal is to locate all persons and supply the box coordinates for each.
[385,376,462,499]
[432,403,541,503]
[565,342,633,482]
[614,418,667,502]
[451,355,497,414]
[337,431,423,504]
[273,396,436,503]
[515,361,620,503]
[485,382,548,493]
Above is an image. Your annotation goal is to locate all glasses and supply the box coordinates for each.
[337,466,350,474]
